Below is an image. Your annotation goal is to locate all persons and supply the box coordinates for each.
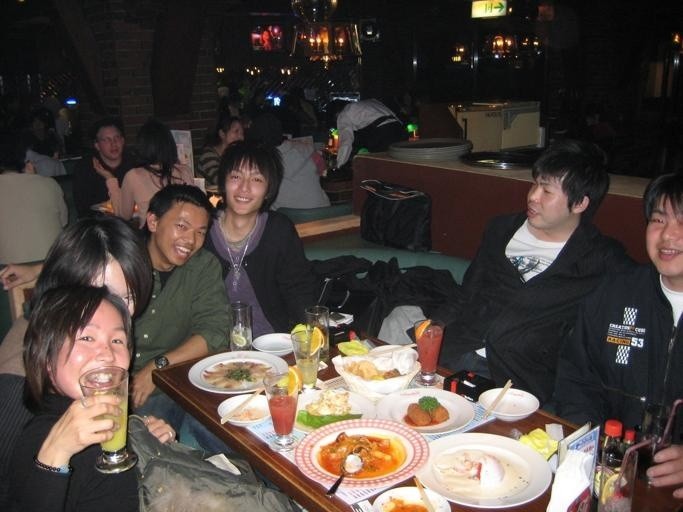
[2,88,418,511]
[437,138,628,410]
[552,170,683,499]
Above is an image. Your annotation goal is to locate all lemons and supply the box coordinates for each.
[310,326,325,357]
[415,320,431,339]
[287,366,302,396]
[232,335,247,347]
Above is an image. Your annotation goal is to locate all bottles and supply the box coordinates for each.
[625,429,637,448]
[598,419,624,472]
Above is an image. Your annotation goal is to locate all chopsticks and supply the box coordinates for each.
[413,478,437,512]
[481,381,514,419]
[220,390,260,424]
[371,342,417,357]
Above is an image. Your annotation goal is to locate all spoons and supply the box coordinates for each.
[325,454,363,498]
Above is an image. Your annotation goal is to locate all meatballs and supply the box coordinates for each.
[407,404,449,426]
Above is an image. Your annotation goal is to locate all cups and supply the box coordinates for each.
[78,366,138,477]
[636,404,676,477]
[414,318,445,390]
[600,445,638,512]
[227,301,254,350]
[262,304,331,451]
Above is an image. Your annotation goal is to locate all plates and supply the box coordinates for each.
[373,486,451,512]
[292,386,553,509]
[461,151,543,171]
[189,352,289,395]
[390,137,473,162]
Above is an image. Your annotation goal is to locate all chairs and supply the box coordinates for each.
[4,278,40,323]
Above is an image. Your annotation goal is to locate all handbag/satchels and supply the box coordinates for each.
[309,255,464,343]
[359,179,434,253]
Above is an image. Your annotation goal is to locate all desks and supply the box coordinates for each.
[351,154,653,267]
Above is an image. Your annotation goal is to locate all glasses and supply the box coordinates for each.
[95,136,123,144]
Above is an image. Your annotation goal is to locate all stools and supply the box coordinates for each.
[276,206,360,238]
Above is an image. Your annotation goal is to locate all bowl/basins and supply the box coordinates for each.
[252,332,296,356]
[218,392,271,428]
[371,345,418,362]
[336,356,421,398]
[479,387,540,424]
